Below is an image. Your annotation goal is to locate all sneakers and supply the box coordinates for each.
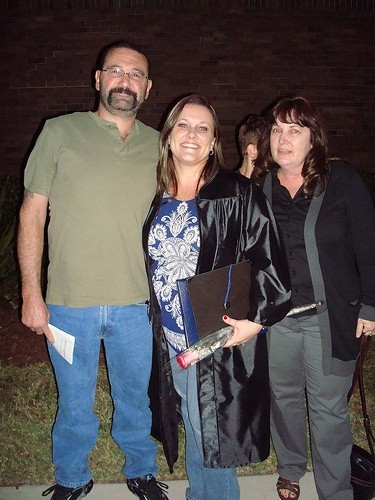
[127,474,171,500]
[41,479,94,500]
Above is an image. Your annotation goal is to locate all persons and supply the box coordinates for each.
[142,95,292,500]
[18,43,170,500]
[233,115,270,179]
[249,95,375,500]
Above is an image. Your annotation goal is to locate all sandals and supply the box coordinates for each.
[275,475,299,500]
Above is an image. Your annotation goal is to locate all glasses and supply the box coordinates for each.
[101,68,149,83]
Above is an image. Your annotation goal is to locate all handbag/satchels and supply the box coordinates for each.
[350,444,375,500]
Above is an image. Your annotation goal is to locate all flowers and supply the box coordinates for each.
[160,299,325,378]
